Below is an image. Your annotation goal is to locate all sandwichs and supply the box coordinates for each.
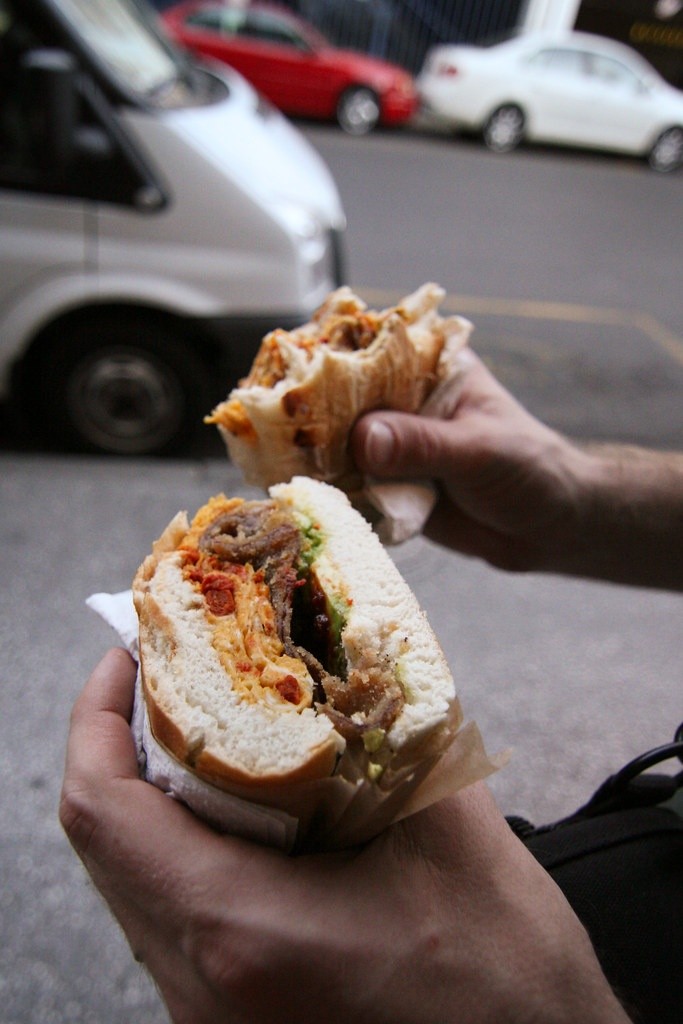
[125,283,478,864]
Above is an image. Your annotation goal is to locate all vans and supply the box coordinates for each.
[0,0,351,458]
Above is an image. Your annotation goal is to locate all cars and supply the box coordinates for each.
[153,0,420,136]
[413,27,683,174]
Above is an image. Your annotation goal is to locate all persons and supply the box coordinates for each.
[54,334,679,1024]
